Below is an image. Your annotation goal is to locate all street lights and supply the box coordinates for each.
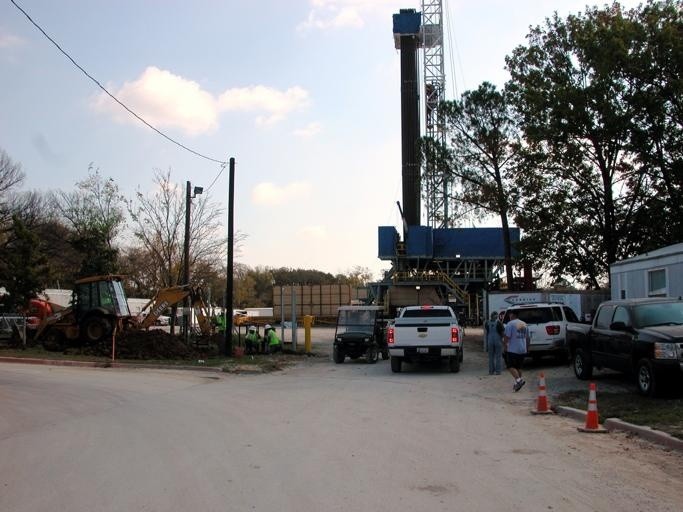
[416,286,421,306]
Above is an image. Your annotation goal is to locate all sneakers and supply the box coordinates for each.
[512,378,525,392]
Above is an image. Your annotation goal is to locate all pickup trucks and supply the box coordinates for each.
[566,297,683,398]
[386,306,465,372]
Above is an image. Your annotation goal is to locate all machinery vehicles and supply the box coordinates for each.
[34,273,217,355]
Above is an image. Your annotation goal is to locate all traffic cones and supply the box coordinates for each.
[577,383,609,432]
[531,372,553,414]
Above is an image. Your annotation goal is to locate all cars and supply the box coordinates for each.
[502,302,592,365]
[138,312,171,326]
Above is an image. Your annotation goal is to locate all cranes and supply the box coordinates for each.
[422,0,448,271]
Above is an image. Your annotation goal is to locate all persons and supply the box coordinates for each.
[244,325,256,353]
[218,311,224,335]
[260,324,279,353]
[502,309,530,393]
[484,311,504,375]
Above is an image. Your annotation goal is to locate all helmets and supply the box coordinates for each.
[264,324,272,330]
[249,325,257,330]
